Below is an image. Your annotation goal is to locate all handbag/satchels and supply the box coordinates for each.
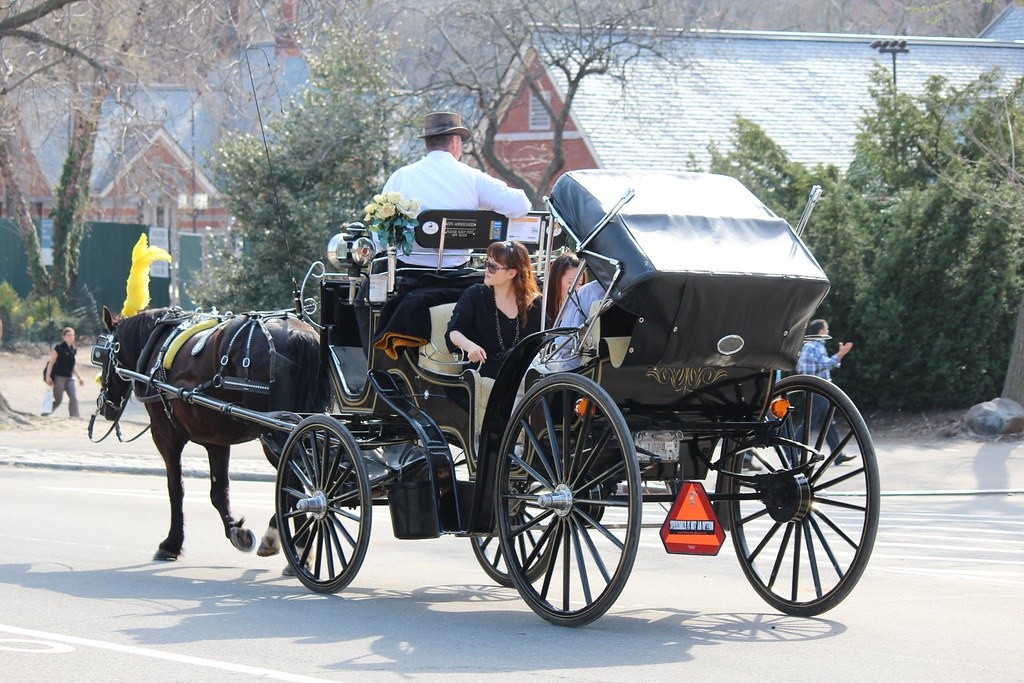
[41,385,54,414]
[43,361,55,384]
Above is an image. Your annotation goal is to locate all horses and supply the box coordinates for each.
[96,306,319,575]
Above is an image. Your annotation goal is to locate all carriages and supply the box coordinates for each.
[87,164,881,629]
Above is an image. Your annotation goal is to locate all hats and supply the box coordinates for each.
[416,112,471,141]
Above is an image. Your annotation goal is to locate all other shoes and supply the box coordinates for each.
[742,461,762,472]
[834,455,857,465]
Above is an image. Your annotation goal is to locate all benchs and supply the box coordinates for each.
[416,300,463,378]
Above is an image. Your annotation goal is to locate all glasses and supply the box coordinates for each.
[482,259,508,274]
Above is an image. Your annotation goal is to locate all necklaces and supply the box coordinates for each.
[495,307,519,352]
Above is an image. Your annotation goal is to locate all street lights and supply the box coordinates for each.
[870,40,910,191]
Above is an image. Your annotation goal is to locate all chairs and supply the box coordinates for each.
[367,208,566,305]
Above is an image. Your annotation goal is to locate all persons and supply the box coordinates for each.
[444,241,612,463]
[42,327,84,420]
[741,319,856,471]
[354,112,532,362]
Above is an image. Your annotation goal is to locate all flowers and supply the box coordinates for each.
[362,189,421,257]
